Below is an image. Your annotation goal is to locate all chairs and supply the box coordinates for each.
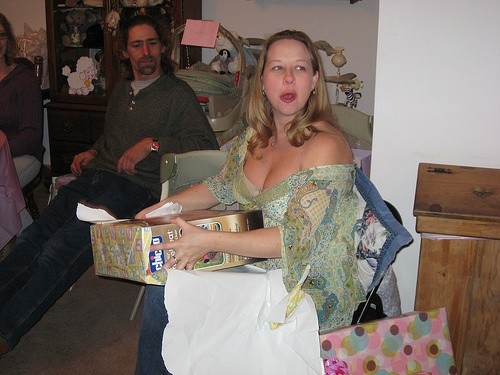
[21,55,43,224]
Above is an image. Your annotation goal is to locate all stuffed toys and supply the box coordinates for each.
[217,49,232,75]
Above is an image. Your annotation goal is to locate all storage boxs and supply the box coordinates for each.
[318,307,460,375]
[90,211,262,285]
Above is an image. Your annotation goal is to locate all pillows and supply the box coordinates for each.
[351,164,415,309]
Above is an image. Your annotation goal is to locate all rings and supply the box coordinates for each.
[173,255,178,263]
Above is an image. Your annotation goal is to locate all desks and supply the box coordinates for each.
[412,162,500,375]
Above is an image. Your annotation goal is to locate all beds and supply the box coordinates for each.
[159,39,374,202]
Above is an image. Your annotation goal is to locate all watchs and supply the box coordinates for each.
[150,136,160,153]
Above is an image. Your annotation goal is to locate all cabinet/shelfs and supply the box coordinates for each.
[44,0,203,178]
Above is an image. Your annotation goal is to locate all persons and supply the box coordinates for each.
[0,15,219,357]
[0,13,46,257]
[135,31,366,375]
